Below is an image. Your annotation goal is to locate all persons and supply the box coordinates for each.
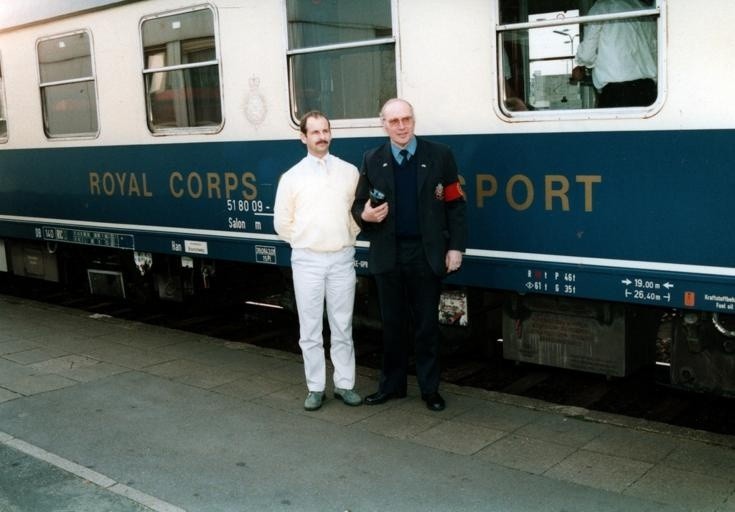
[503,49,512,100]
[273,109,363,411]
[351,99,467,411]
[573,1,658,108]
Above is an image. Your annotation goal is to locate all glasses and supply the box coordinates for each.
[388,118,411,128]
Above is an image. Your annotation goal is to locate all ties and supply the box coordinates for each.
[399,147,409,165]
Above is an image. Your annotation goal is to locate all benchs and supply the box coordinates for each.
[148,88,221,128]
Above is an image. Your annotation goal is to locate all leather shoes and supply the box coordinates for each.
[421,391,445,412]
[364,392,392,404]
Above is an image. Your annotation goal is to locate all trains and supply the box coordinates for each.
[0,0,735,397]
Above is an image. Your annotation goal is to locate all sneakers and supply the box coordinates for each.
[333,386,363,406]
[304,391,326,410]
